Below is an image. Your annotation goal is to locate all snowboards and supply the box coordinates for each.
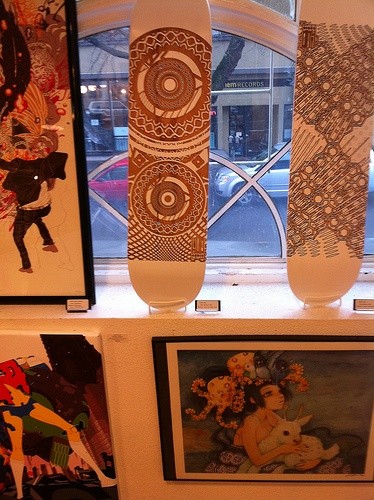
[129,1,209,309]
[288,0,374,308]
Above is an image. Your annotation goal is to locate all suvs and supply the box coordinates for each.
[82,99,131,123]
[214,142,373,207]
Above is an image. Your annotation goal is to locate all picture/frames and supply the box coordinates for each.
[152,334,374,484]
[0,0,95,308]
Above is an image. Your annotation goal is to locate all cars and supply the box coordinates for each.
[89,150,129,212]
